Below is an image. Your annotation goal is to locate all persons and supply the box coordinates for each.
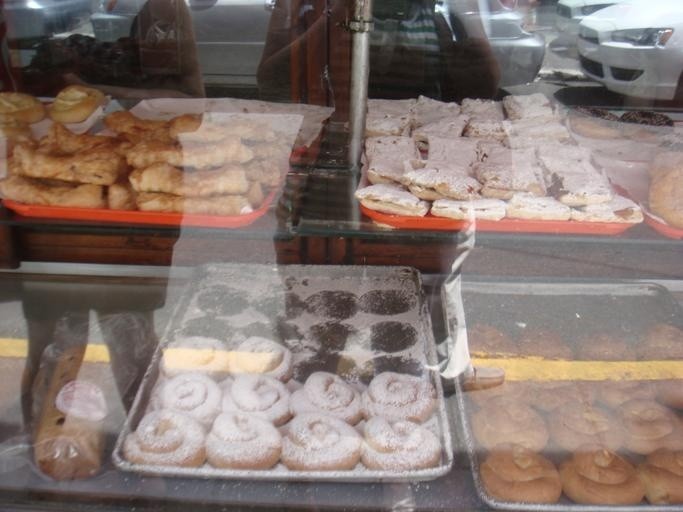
[257,1,506,394]
[1,0,207,442]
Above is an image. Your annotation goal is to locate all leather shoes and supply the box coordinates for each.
[442,364,506,393]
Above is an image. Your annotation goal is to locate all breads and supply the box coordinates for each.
[573,104,683,231]
[36,308,151,481]
[467,324,683,505]
[353,91,645,231]
[0,84,283,214]
[123,286,442,481]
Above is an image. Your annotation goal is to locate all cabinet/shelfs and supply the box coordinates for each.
[0,114,681,510]
